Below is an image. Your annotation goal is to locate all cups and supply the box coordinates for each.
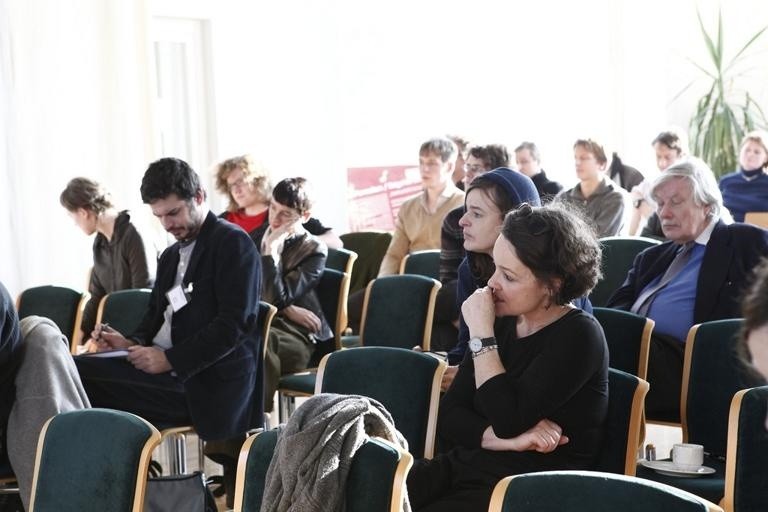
[672,444,704,470]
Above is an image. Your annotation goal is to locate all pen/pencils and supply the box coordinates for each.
[95,323,109,342]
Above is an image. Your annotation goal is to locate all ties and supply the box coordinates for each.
[630,242,697,317]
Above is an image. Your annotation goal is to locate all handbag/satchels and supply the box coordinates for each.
[141,470,219,512]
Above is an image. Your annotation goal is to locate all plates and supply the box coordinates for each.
[641,461,715,478]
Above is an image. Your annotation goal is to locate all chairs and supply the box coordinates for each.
[1,212,768,512]
